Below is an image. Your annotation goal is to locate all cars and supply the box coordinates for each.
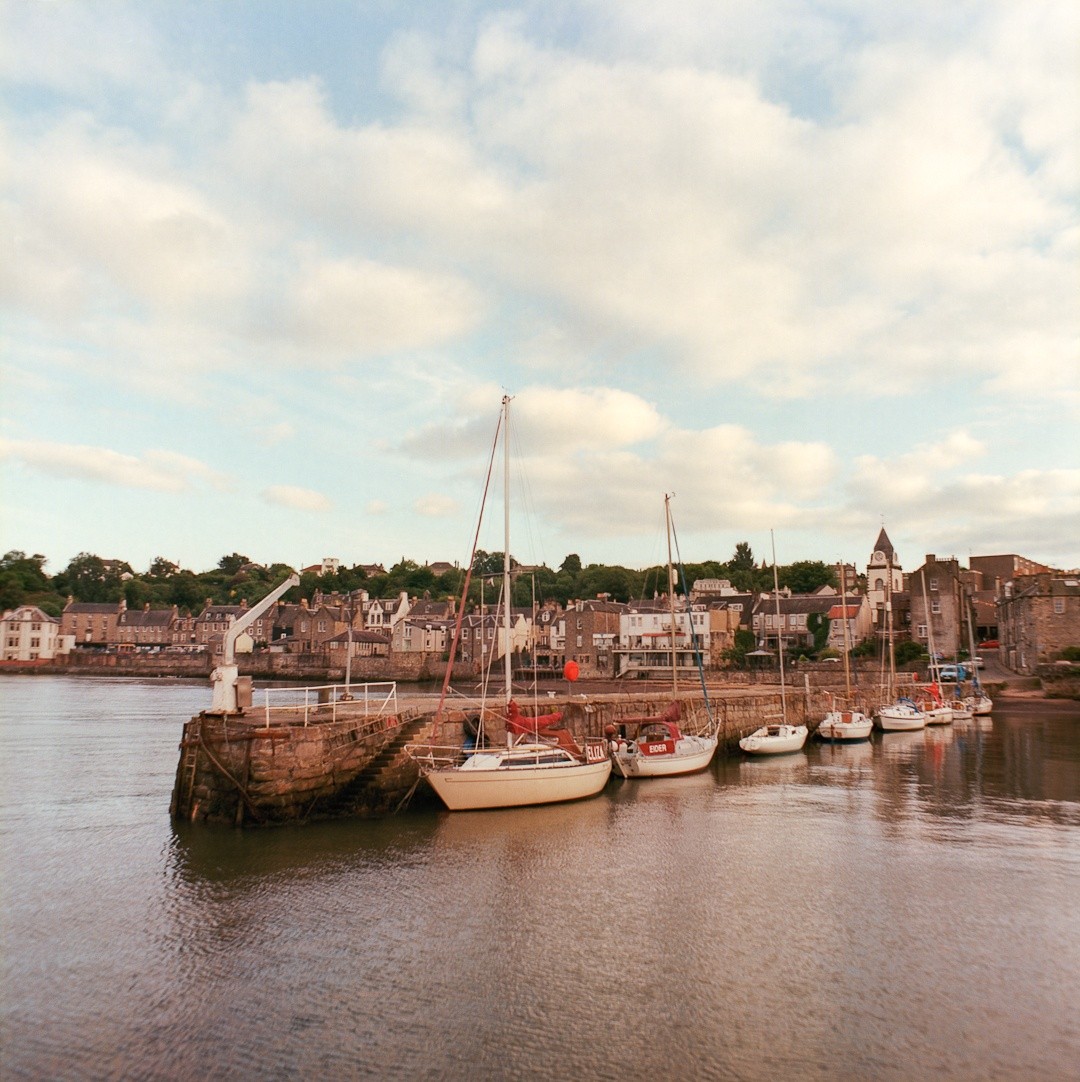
[939,657,986,682]
[979,639,1000,649]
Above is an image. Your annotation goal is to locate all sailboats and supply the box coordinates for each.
[738,527,808,754]
[604,492,722,779]
[818,560,874,739]
[871,559,925,731]
[404,381,614,814]
[914,567,993,724]
